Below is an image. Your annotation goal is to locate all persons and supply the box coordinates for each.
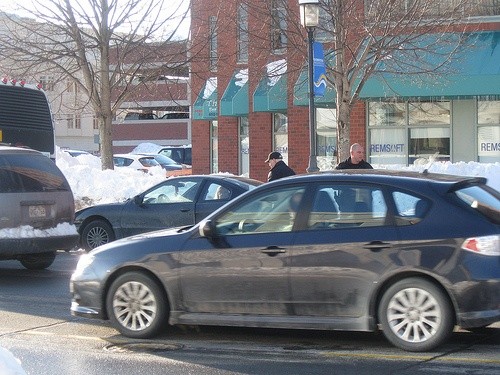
[281,192,318,232]
[332,143,377,220]
[264,151,297,208]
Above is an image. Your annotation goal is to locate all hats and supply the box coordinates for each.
[264,152,283,163]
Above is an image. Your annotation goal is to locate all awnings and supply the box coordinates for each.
[348,30,500,99]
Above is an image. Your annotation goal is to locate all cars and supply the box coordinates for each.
[143,154,192,179]
[113,153,167,181]
[73,173,279,255]
[68,167,500,353]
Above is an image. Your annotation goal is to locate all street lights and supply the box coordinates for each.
[297,0,321,173]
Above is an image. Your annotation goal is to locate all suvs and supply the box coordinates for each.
[0,142,82,271]
